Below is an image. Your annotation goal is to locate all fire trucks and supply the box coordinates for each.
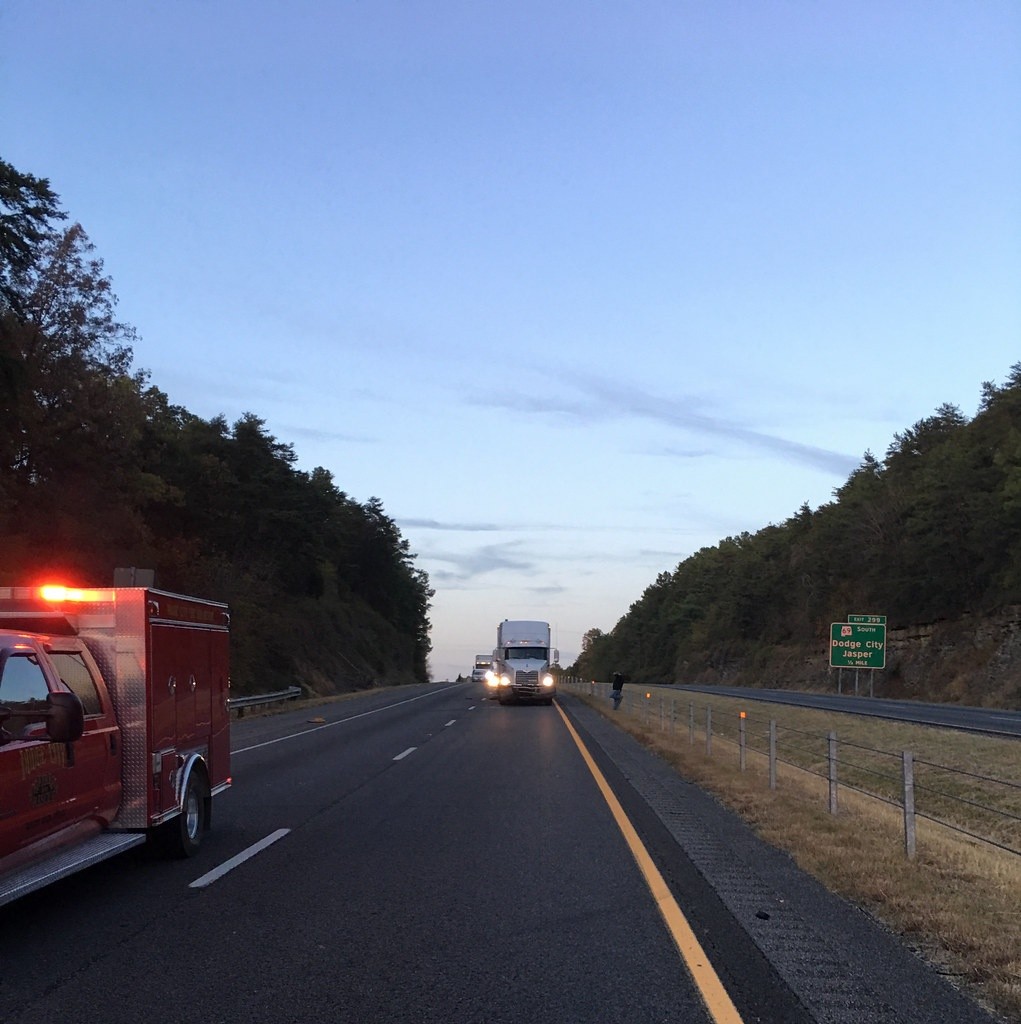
[0,584,235,907]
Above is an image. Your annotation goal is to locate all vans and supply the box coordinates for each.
[472,670,486,682]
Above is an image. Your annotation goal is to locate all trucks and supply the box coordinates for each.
[475,655,493,673]
[493,621,559,706]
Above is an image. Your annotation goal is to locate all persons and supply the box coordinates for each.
[609,671,624,710]
